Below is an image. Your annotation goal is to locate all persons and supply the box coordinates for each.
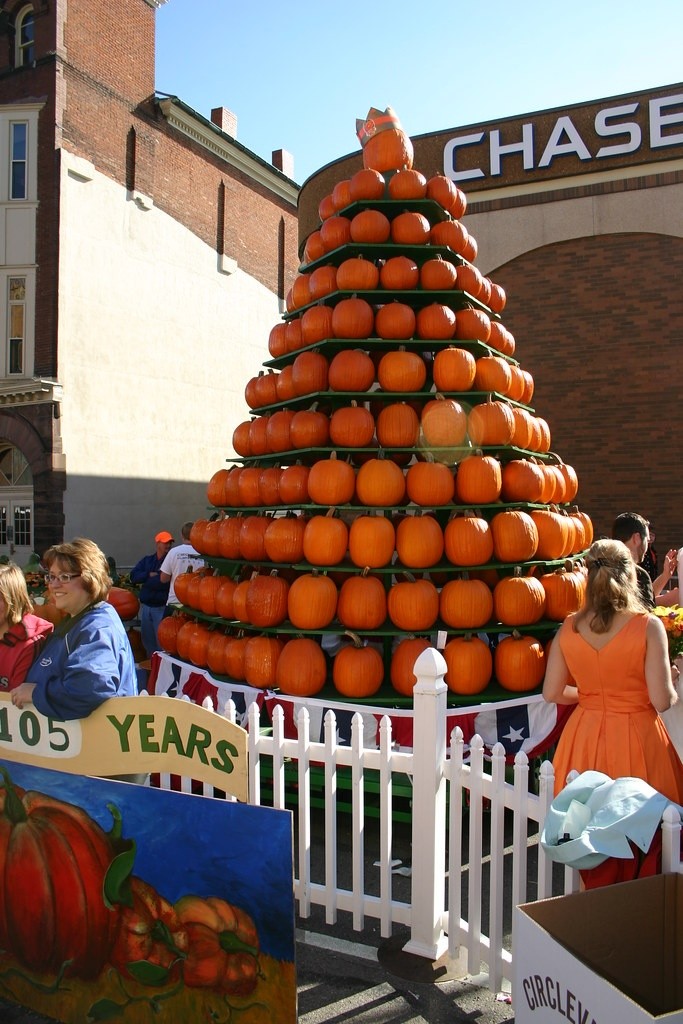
[637,528,659,583]
[11,537,148,785]
[612,511,656,612]
[0,555,16,565]
[0,562,54,692]
[543,540,682,808]
[651,548,683,610]
[130,531,175,659]
[160,522,204,621]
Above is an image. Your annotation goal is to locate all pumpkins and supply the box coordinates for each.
[159,130,599,699]
[25,572,144,649]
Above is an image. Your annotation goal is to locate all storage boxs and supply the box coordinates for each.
[510,872,683,1024]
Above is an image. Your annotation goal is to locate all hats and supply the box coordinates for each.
[154,531,175,543]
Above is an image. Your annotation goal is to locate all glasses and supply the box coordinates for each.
[44,574,81,584]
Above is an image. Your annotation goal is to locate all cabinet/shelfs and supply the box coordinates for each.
[168,169,596,704]
